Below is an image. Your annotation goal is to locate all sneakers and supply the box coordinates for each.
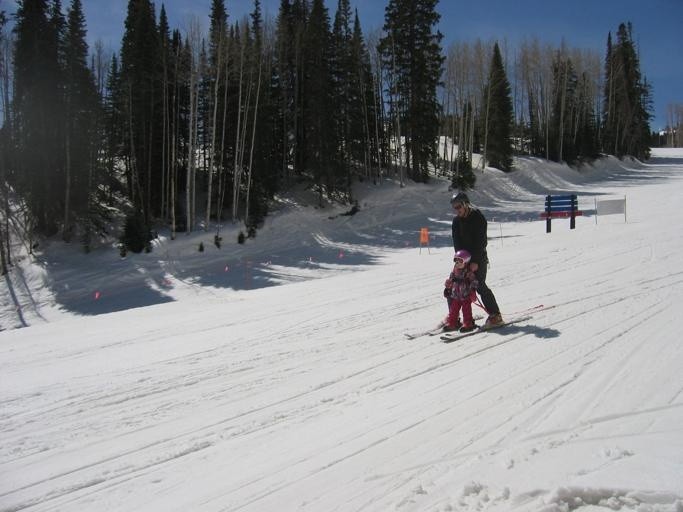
[485,315,504,326]
[442,323,461,332]
[460,323,476,331]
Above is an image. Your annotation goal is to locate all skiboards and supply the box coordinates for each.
[404,311,532,341]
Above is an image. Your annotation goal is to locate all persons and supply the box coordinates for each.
[450,192,503,325]
[443,249,479,332]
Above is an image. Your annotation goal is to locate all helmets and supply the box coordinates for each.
[453,249,472,263]
[449,192,470,204]
